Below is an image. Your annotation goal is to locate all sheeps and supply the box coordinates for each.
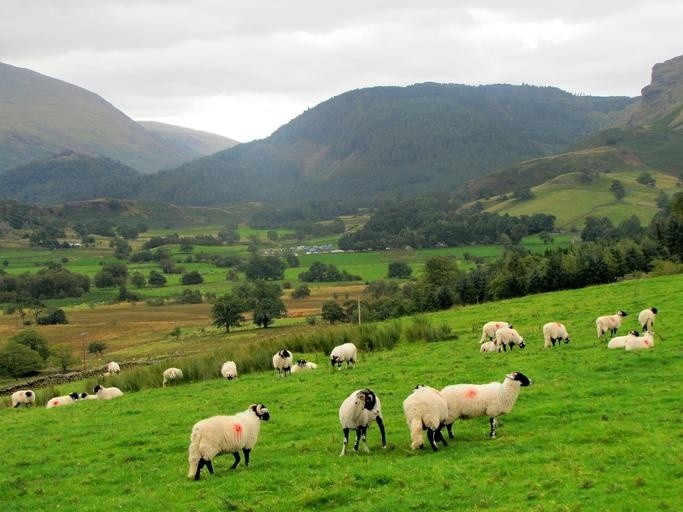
[10,390,36,408]
[187,402,271,480]
[596,307,660,351]
[220,360,238,380]
[542,322,570,349]
[439,371,534,439]
[162,367,183,388]
[46,384,124,409]
[479,320,526,353]
[99,361,121,378]
[330,342,357,371]
[272,349,318,378]
[338,388,387,458]
[402,384,448,452]
